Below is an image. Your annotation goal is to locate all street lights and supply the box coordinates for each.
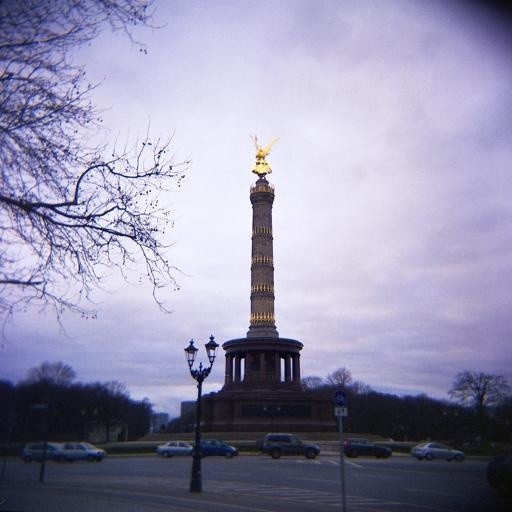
[183,334,220,494]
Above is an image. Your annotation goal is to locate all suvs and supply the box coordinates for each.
[256,432,322,459]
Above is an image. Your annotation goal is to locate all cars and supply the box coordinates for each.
[410,442,465,462]
[343,438,394,460]
[60,441,106,462]
[191,439,239,459]
[24,442,74,463]
[157,440,198,459]
